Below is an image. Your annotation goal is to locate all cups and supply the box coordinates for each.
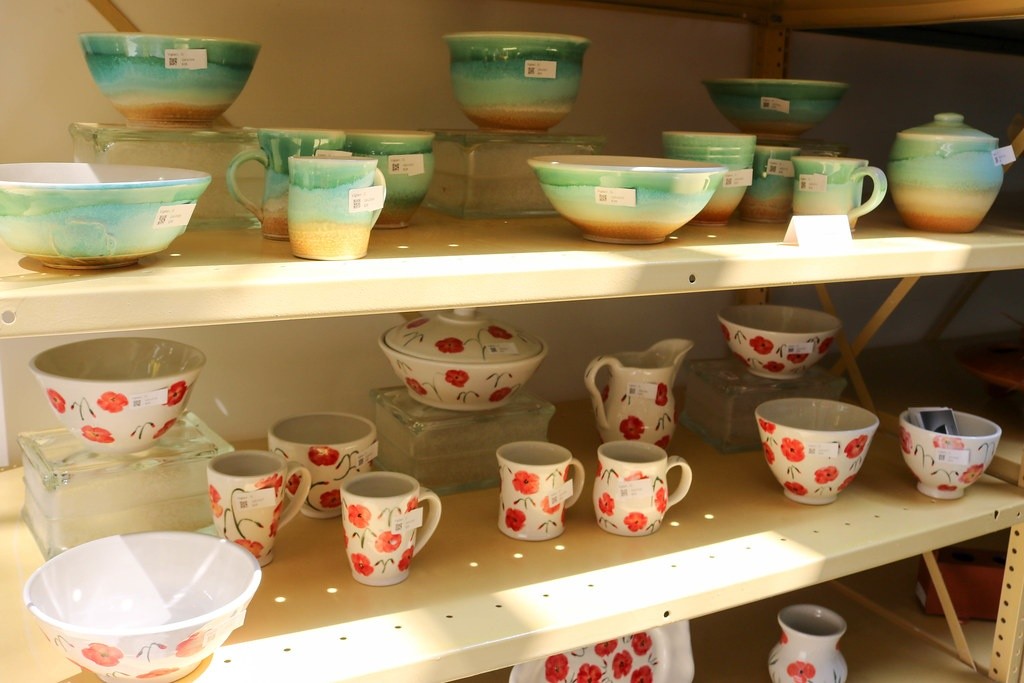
[659,128,757,226]
[287,155,386,261]
[223,132,346,242]
[340,472,443,586]
[495,440,584,542]
[790,156,886,234]
[208,451,312,569]
[594,441,692,538]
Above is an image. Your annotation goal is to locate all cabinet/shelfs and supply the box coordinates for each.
[1,4,1024,683]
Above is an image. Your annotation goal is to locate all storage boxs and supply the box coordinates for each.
[917,549,1005,620]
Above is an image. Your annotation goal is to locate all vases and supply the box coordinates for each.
[767,604,849,683]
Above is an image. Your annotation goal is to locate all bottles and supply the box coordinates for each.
[769,604,848,683]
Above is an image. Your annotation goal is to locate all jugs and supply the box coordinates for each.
[583,338,694,451]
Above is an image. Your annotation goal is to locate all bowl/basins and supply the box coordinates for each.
[4,32,1014,683]
